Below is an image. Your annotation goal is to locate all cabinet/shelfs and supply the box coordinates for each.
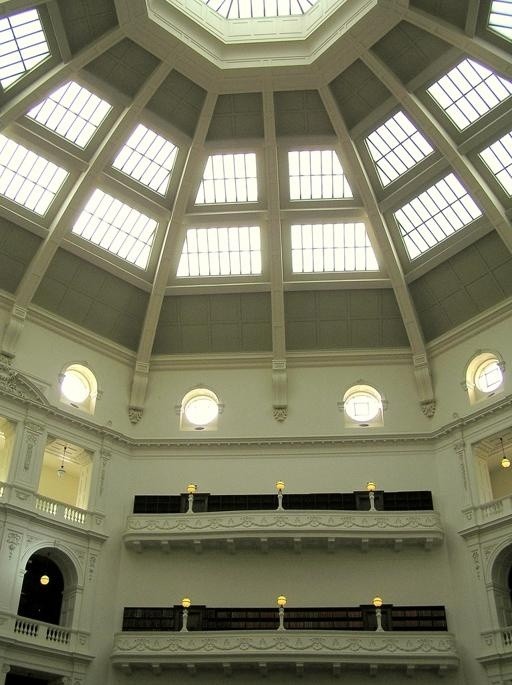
[133,490,433,513]
[123,605,447,630]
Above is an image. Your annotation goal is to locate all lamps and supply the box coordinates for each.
[40,552,51,586]
[500,437,510,467]
[181,596,196,631]
[373,596,384,632]
[367,481,377,510]
[186,482,196,513]
[275,479,285,510]
[57,446,67,478]
[277,594,288,631]
